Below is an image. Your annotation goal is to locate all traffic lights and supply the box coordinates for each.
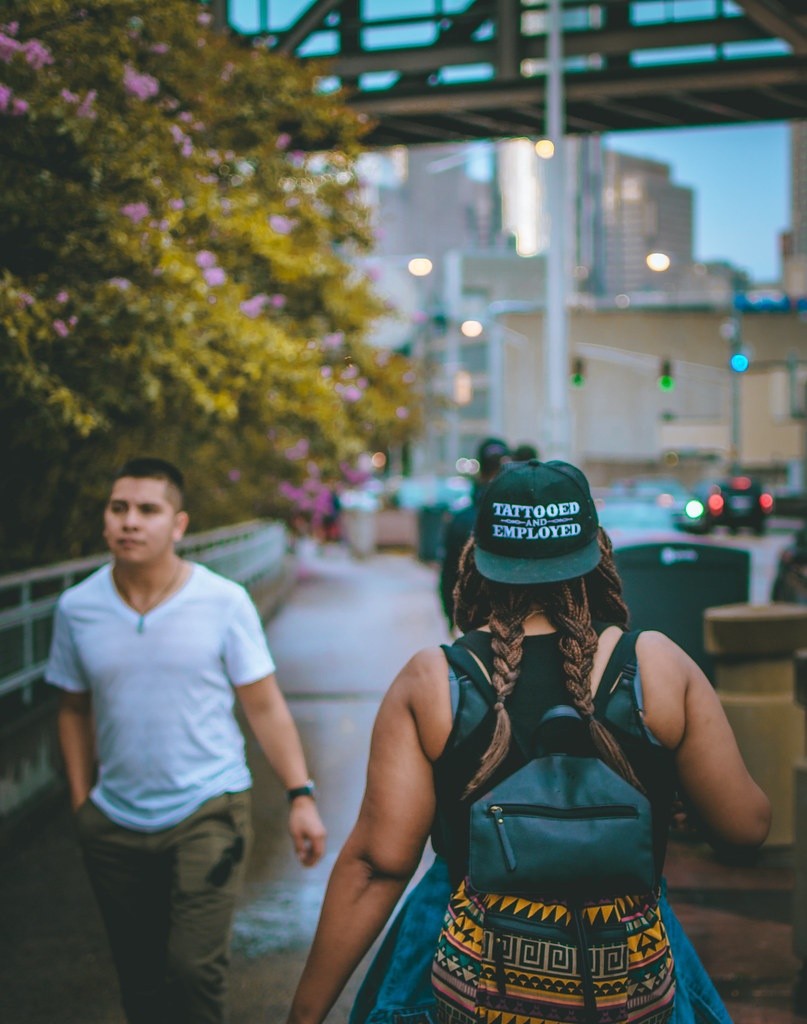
[573,355,584,389]
[730,353,750,375]
[658,356,675,391]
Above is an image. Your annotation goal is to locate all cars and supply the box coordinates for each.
[585,468,800,686]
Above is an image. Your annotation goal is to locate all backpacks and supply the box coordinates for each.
[430,623,676,1024]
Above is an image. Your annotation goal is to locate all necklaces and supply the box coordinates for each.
[114,551,185,636]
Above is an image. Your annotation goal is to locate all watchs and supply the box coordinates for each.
[287,780,318,802]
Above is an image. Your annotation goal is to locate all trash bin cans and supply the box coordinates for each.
[703,603,807,850]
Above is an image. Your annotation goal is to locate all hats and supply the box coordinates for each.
[474,459,602,584]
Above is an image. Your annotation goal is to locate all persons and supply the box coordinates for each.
[438,437,540,642]
[47,458,324,1024]
[286,457,772,1024]
